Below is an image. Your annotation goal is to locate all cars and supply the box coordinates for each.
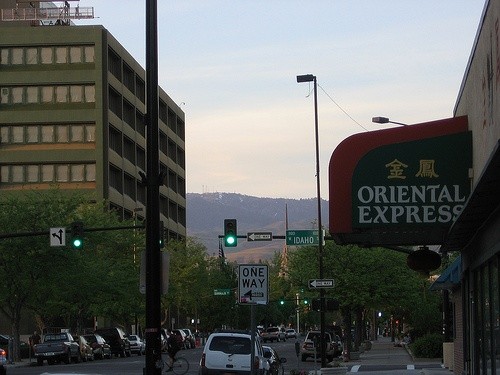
[161,328,180,353]
[131,335,146,356]
[199,333,272,375]
[301,330,342,363]
[84,333,112,360]
[94,328,132,358]
[172,329,192,350]
[262,345,287,375]
[183,329,196,349]
[72,336,94,362]
[0,349,7,375]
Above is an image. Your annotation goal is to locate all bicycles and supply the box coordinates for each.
[160,347,189,375]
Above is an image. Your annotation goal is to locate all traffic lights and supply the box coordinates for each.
[280,301,284,305]
[304,300,308,305]
[71,221,84,250]
[224,219,237,248]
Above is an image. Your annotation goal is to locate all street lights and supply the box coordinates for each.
[297,74,327,368]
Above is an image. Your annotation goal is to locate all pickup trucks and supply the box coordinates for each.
[33,333,81,366]
[256,326,296,343]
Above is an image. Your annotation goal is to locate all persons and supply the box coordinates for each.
[160,327,179,372]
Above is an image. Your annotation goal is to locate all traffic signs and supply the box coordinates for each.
[247,232,272,241]
[239,264,268,305]
[308,279,334,288]
